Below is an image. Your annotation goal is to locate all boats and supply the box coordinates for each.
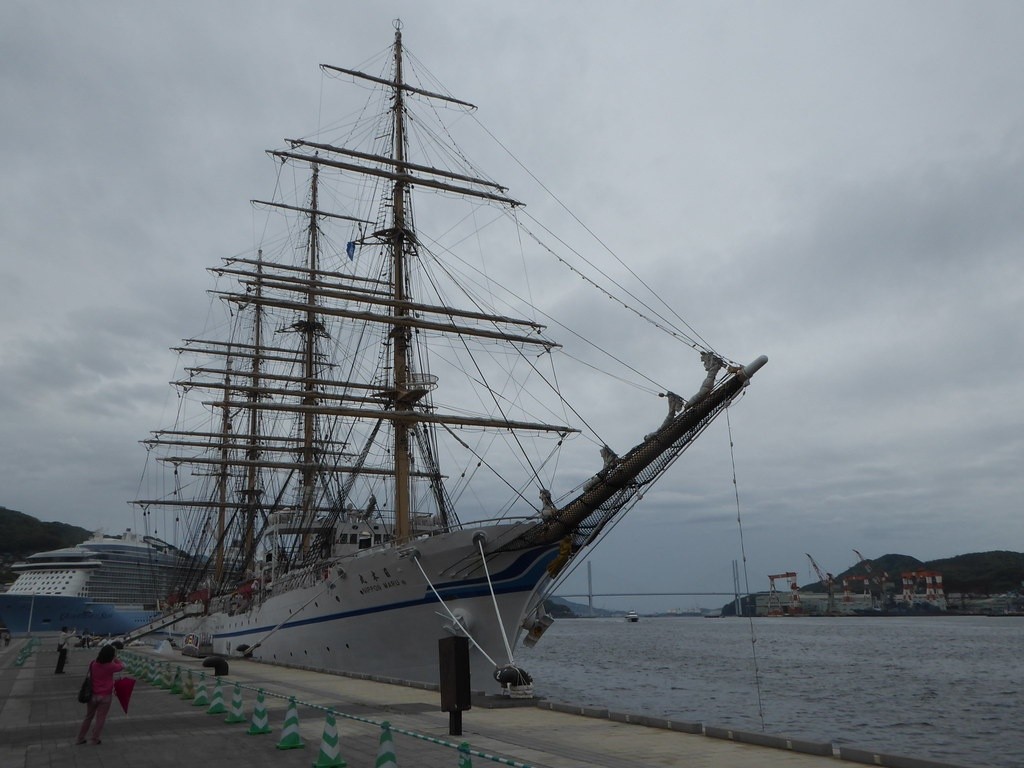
[624,609,640,623]
[0,522,245,638]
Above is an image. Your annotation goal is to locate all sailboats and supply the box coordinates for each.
[110,16,771,700]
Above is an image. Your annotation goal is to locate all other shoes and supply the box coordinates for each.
[76,738,87,745]
[92,740,101,745]
[55,671,65,674]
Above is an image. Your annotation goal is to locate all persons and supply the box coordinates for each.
[82,631,90,648]
[55,626,76,674]
[75,644,125,745]
[5,630,11,646]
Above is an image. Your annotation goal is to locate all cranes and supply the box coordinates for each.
[768,548,946,616]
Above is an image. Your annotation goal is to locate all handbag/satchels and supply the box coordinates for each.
[79,677,92,703]
[57,643,63,651]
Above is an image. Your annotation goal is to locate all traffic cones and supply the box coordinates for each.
[374,721,399,768]
[225,681,248,724]
[276,696,306,749]
[169,665,184,695]
[191,671,211,707]
[151,661,165,686]
[458,741,472,768]
[160,662,173,690]
[15,635,41,666]
[247,687,273,735]
[115,648,156,683]
[311,705,348,768]
[206,677,228,714]
[180,669,195,700]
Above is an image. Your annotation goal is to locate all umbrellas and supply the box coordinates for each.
[114,670,136,715]
